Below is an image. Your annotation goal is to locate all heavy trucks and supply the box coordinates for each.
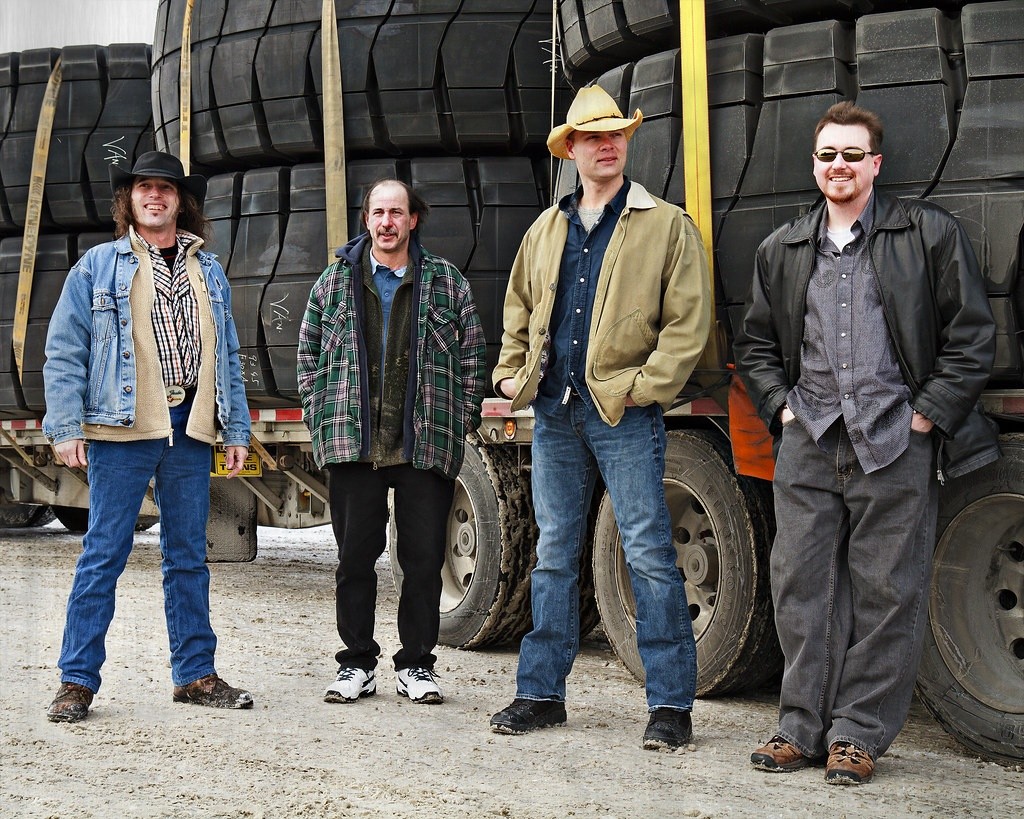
[3,2,1023,768]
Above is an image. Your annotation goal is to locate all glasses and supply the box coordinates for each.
[812,146,876,163]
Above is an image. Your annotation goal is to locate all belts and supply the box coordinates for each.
[164,385,198,407]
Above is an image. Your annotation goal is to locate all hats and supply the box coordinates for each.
[546,85,643,160]
[107,151,207,215]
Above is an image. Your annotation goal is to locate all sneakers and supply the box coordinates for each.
[323,667,376,703]
[750,735,828,773]
[173,673,254,709]
[643,706,693,750]
[46,681,93,723]
[824,740,874,785]
[490,698,568,735]
[396,666,443,704]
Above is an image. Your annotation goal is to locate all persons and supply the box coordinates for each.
[735,99,996,783]
[42,150,253,721]
[296,178,492,703]
[491,85,716,753]
